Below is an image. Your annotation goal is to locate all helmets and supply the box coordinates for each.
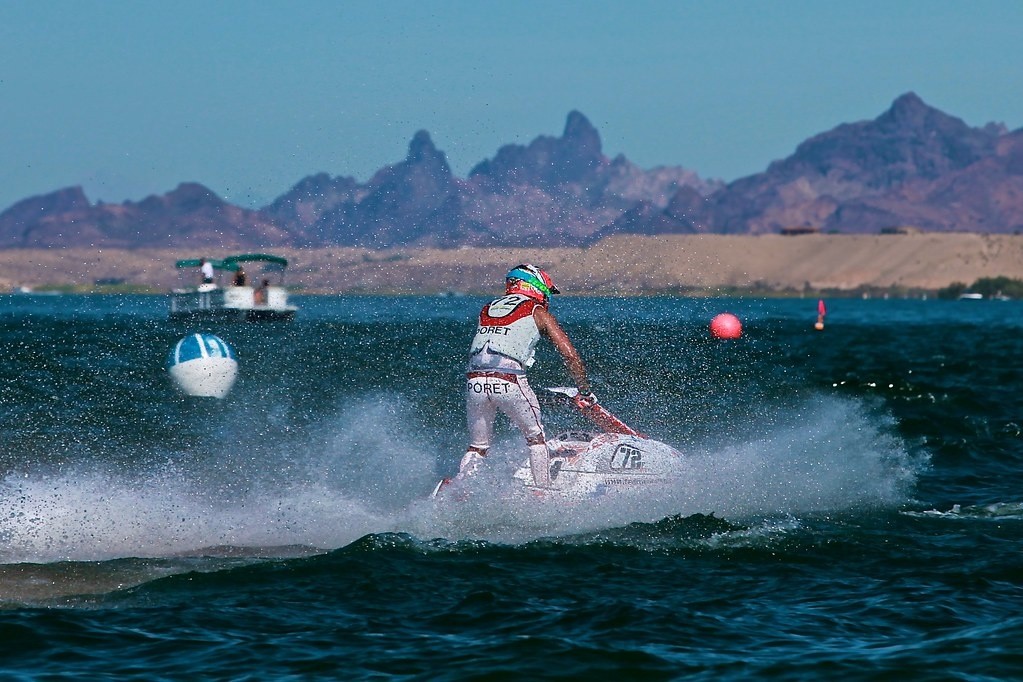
[506,263,560,311]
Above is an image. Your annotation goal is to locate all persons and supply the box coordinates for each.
[233,265,244,286]
[201,257,213,283]
[459,262,598,490]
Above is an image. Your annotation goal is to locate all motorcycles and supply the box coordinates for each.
[426,386,693,504]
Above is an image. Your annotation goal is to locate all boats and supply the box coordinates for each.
[167,253,300,325]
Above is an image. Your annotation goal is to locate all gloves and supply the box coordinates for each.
[576,388,598,409]
